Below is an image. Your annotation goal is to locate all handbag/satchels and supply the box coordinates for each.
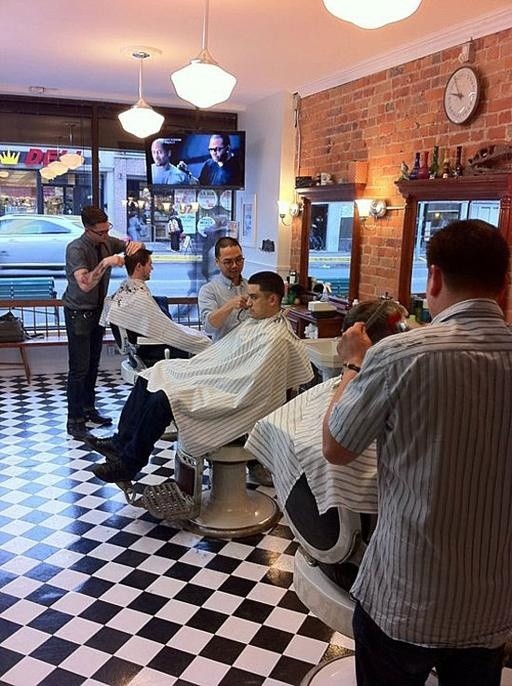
[0,312,45,343]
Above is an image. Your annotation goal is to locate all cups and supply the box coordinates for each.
[320,171,332,188]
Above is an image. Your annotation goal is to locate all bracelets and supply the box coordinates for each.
[341,362,360,372]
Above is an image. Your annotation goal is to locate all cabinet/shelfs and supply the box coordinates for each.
[279,305,346,338]
[298,338,344,393]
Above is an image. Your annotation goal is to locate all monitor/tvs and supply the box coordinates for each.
[145,129,247,190]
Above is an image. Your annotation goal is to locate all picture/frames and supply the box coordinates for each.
[238,192,257,249]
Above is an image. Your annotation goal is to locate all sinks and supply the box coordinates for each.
[299,336,345,367]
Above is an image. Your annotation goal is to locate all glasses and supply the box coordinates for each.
[208,146,224,152]
[221,257,245,270]
[89,223,114,236]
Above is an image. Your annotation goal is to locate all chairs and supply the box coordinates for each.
[116,381,281,538]
[255,417,441,685]
[103,297,211,442]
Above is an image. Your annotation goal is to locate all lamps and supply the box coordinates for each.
[275,199,300,228]
[354,197,387,231]
[39,123,85,180]
[321,0,423,31]
[169,0,238,109]
[117,50,165,140]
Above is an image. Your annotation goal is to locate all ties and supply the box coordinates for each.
[97,245,104,308]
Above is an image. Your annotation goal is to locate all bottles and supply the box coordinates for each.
[281,279,298,306]
[352,299,359,307]
[400,143,465,182]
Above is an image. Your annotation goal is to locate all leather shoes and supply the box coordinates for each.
[92,461,135,483]
[84,436,120,461]
[67,422,94,439]
[84,410,112,424]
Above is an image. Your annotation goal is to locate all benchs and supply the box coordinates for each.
[0,299,203,386]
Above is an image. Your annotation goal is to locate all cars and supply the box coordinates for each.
[0,212,132,273]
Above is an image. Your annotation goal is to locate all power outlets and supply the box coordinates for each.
[107,345,115,356]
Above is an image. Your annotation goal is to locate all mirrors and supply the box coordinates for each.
[393,177,512,318]
[295,183,362,311]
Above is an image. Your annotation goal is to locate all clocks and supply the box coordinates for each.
[443,66,481,125]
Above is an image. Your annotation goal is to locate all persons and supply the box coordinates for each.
[177,134,241,186]
[320,219,512,685]
[83,271,284,484]
[341,299,407,344]
[62,206,146,439]
[124,250,193,358]
[151,139,200,186]
[167,211,183,253]
[202,215,230,281]
[128,210,143,242]
[197,237,274,487]
